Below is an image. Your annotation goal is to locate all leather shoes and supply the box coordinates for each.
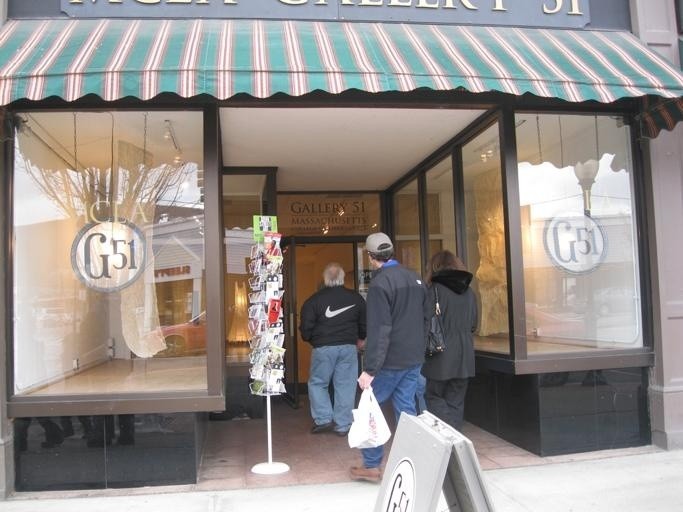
[328,429,348,438]
[311,422,337,434]
[348,467,382,482]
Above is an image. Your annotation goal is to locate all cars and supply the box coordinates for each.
[594,284,635,316]
[31,307,69,326]
[145,303,208,362]
[524,302,587,340]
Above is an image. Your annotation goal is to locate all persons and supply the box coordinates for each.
[84,416,137,448]
[13,418,66,453]
[345,232,435,486]
[420,249,483,434]
[297,261,366,437]
[57,416,94,441]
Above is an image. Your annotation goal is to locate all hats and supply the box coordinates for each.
[364,231,394,253]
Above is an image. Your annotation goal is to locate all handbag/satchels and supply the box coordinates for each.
[425,311,446,360]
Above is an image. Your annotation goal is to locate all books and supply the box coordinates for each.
[250,213,288,398]
